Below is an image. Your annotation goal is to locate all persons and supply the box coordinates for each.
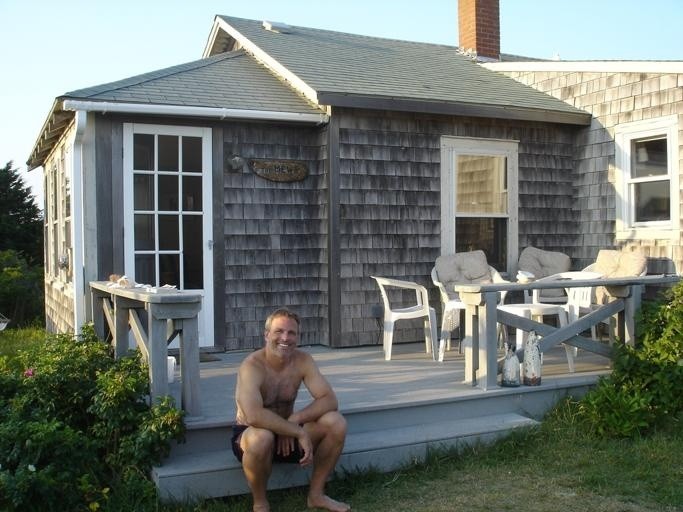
[230,308,349,510]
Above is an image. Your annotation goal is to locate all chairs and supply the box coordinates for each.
[430,247,511,364]
[367,274,438,362]
[532,268,609,358]
[513,245,569,366]
[579,246,650,344]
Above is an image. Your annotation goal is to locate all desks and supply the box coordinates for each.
[435,298,574,373]
